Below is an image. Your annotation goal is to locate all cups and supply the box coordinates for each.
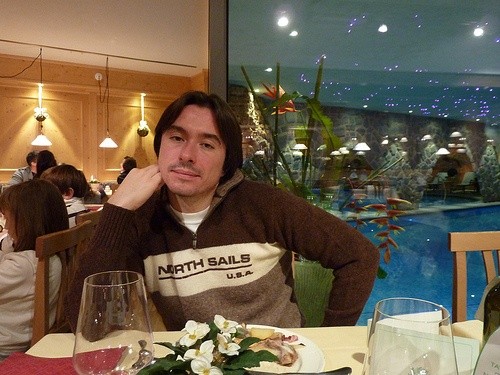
[72,271,154,375]
[362,297,458,375]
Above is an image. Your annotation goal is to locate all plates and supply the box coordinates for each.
[181,324,325,375]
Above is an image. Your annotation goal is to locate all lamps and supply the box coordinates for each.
[99,131,118,148]
[31,125,52,146]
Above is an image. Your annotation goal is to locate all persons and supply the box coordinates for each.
[0,178,70,364]
[9,150,38,186]
[117,158,137,184]
[32,150,57,178]
[0,164,90,252]
[120,156,133,174]
[66,90,380,343]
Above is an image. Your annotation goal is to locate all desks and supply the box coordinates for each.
[0,326,368,375]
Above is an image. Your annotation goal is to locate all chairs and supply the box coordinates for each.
[30,220,93,347]
[448,231,500,324]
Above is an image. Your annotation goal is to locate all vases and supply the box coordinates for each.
[291,261,335,328]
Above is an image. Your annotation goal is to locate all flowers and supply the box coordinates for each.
[137,314,279,375]
[241,56,412,279]
